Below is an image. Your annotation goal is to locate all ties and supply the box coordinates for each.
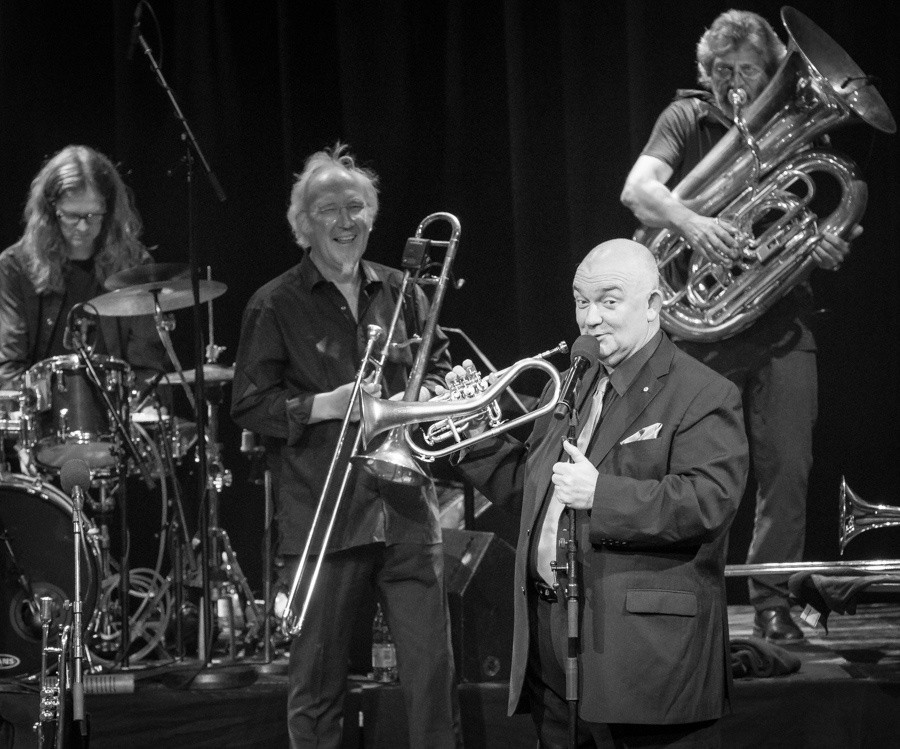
[534,376,611,593]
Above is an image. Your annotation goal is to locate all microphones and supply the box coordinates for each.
[63,311,76,350]
[841,77,851,88]
[130,372,163,409]
[554,335,600,420]
[61,459,90,511]
[127,2,143,62]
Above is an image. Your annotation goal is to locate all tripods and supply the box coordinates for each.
[117,383,280,663]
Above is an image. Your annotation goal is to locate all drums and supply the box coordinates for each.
[0,474,100,688]
[15,354,133,468]
[145,366,252,482]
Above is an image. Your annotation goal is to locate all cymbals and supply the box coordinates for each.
[84,278,227,317]
[104,264,192,291]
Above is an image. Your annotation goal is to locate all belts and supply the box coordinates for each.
[531,580,560,603]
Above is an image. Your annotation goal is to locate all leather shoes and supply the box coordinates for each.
[752,606,806,645]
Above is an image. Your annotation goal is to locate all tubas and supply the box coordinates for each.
[630,6,898,345]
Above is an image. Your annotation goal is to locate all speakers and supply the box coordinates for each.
[371,528,518,681]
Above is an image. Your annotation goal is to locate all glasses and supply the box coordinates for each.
[52,200,108,225]
[711,65,766,79]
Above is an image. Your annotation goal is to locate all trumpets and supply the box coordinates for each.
[357,342,569,459]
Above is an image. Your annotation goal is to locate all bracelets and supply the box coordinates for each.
[834,266,839,272]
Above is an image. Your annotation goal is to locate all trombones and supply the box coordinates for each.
[726,475,900,578]
[282,211,461,636]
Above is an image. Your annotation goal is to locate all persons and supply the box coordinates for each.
[230,143,453,749]
[623,11,863,645]
[0,143,157,415]
[434,235,750,749]
